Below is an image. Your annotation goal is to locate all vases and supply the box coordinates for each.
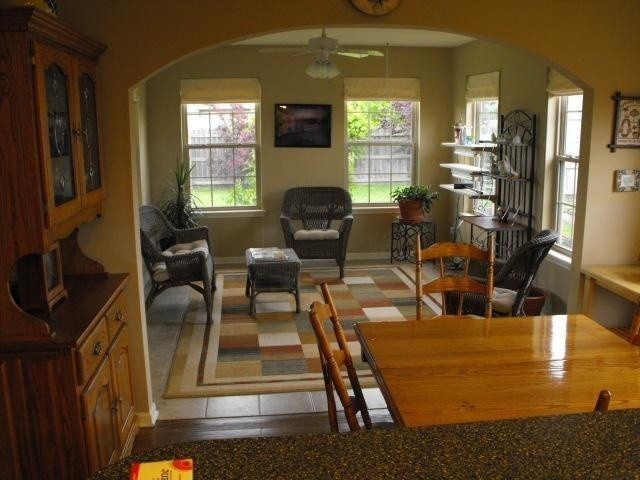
[514,285,548,316]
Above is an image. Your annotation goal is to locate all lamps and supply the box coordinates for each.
[302,56,340,80]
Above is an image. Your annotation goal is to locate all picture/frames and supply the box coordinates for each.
[607,90,640,154]
[274,103,333,149]
[615,168,640,193]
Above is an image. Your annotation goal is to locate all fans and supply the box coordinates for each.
[256,27,386,64]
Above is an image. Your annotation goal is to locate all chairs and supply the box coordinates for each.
[415,234,496,322]
[139,203,219,325]
[279,186,353,279]
[443,229,559,318]
[308,281,398,432]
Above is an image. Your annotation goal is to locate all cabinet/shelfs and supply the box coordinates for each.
[1,7,109,250]
[1,273,142,479]
[459,108,539,278]
[438,138,496,203]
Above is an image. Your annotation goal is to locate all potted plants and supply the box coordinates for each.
[390,184,440,224]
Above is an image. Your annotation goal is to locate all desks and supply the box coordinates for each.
[578,265,640,346]
[354,312,640,427]
[389,222,437,268]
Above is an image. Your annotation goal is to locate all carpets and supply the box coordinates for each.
[162,261,449,400]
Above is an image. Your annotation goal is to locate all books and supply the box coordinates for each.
[128,458,194,480]
